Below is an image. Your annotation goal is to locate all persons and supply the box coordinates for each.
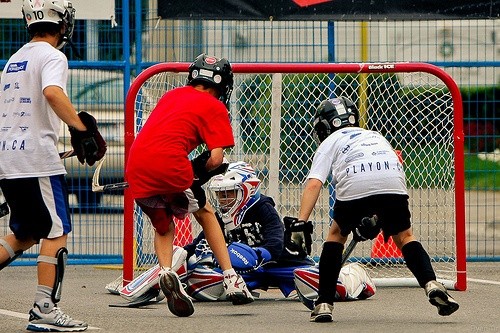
[122,161,375,301]
[126,52,255,318]
[0,0,106,332]
[283,96,459,321]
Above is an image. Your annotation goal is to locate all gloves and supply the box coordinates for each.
[283,216,315,261]
[68,110,108,167]
[350,213,382,242]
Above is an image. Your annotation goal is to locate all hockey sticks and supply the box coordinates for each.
[342,215,378,264]
[92,158,129,193]
[58,150,76,159]
[108,289,160,307]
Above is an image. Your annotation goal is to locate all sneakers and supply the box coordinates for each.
[158,266,195,317]
[310,301,335,323]
[221,273,255,305]
[424,280,459,317]
[26,303,88,332]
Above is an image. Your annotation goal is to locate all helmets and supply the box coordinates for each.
[313,96,360,144]
[210,161,261,231]
[188,54,233,101]
[21,0,76,51]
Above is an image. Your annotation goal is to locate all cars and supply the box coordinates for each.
[50,70,157,207]
